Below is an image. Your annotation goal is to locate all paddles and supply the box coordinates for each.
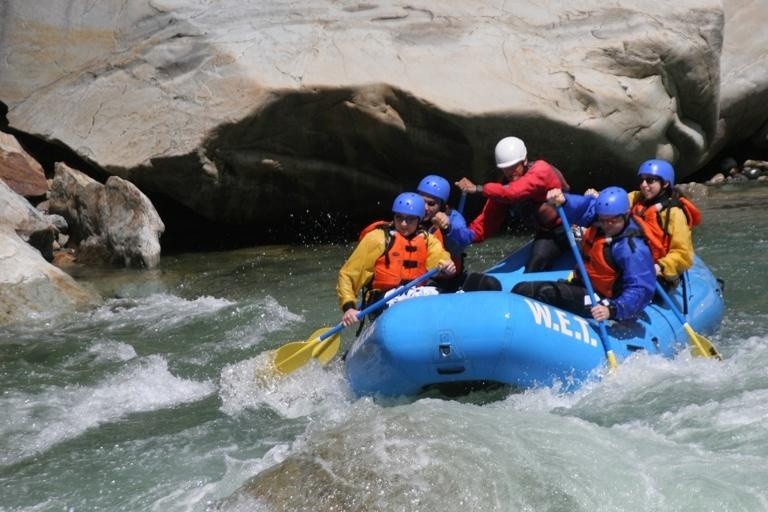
[556,204,619,372]
[653,273,722,362]
[272,259,447,375]
[306,299,363,366]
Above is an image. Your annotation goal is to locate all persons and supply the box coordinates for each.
[584,159,695,305]
[336,192,456,327]
[510,186,656,322]
[454,137,586,274]
[415,174,504,294]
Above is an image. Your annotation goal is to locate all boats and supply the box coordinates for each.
[336,185,729,393]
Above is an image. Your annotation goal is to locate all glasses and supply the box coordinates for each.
[640,176,659,184]
[599,216,618,224]
[395,215,417,224]
[423,198,436,207]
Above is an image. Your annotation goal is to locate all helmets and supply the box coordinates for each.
[392,192,426,225]
[495,137,527,169]
[638,159,675,189]
[417,175,450,204]
[596,187,630,217]
